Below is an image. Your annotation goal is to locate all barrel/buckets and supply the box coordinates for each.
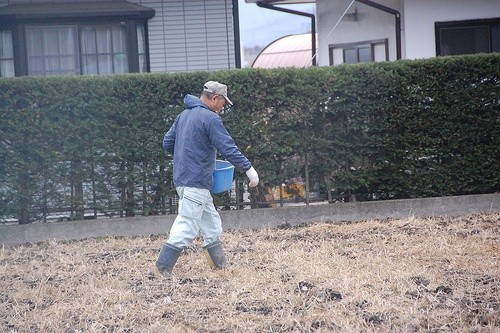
[209,159,235,194]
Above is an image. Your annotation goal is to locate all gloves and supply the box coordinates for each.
[246,166,259,187]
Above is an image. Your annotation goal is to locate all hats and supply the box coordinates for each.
[203,81,233,105]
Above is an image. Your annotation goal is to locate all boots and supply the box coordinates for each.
[202,240,229,270]
[156,242,182,275]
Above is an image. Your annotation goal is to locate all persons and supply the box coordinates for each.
[149,81,259,281]
